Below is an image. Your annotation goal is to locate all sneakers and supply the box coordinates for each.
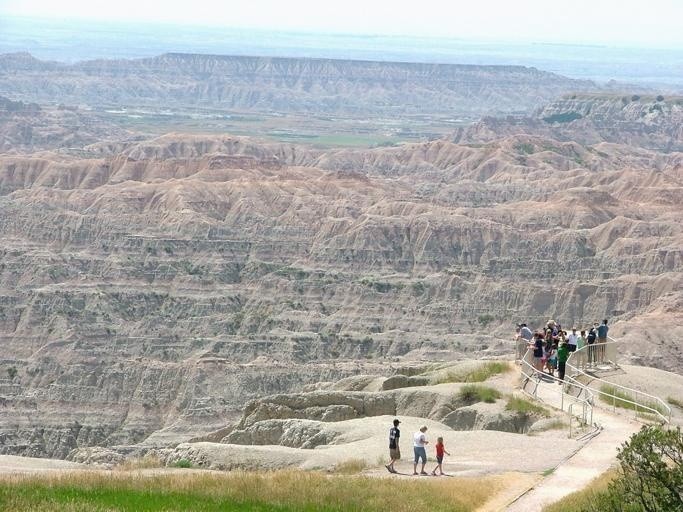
[420,472,427,475]
[431,471,435,476]
[391,470,397,473]
[385,464,392,472]
[412,472,418,475]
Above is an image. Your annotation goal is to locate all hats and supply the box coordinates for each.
[393,420,400,424]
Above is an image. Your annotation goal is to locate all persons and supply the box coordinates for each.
[512,318,609,386]
[412,425,429,475]
[431,437,450,475]
[384,419,402,473]
